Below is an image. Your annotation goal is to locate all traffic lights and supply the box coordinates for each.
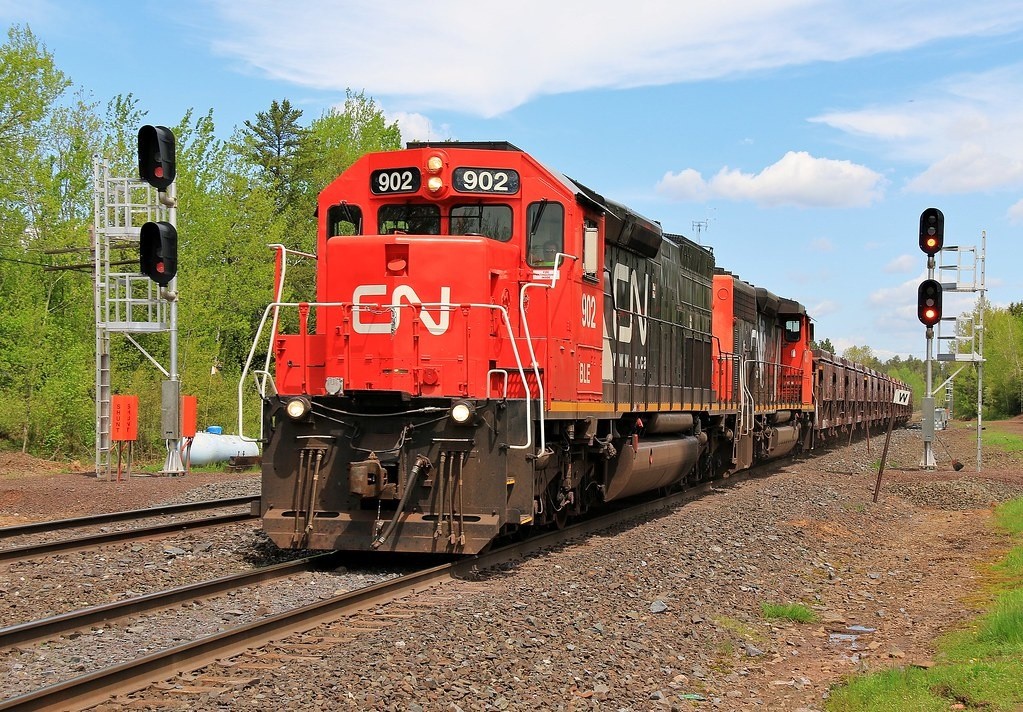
[138,125,175,188]
[139,222,178,284]
[919,207,945,255]
[918,279,943,327]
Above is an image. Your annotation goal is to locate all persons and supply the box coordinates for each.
[532,240,561,266]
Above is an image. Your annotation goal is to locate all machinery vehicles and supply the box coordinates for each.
[934,408,949,431]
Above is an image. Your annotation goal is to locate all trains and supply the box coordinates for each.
[237,141,914,556]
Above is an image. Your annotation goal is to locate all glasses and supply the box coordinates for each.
[544,248,557,255]
